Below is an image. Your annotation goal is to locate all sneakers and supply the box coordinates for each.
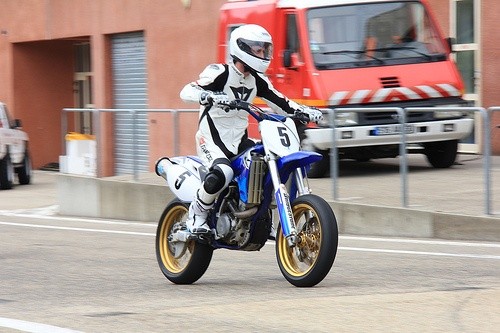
[187,203,211,233]
[269,223,276,240]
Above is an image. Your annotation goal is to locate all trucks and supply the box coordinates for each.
[215,0,476,178]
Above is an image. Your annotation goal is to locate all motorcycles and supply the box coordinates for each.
[155,96,339,288]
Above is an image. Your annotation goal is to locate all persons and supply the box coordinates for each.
[180,22,324,244]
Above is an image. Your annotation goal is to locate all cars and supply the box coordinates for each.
[0,102,31,190]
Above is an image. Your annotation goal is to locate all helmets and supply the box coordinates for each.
[230,24,273,73]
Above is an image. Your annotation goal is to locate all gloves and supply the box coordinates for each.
[297,105,323,124]
[208,91,229,110]
